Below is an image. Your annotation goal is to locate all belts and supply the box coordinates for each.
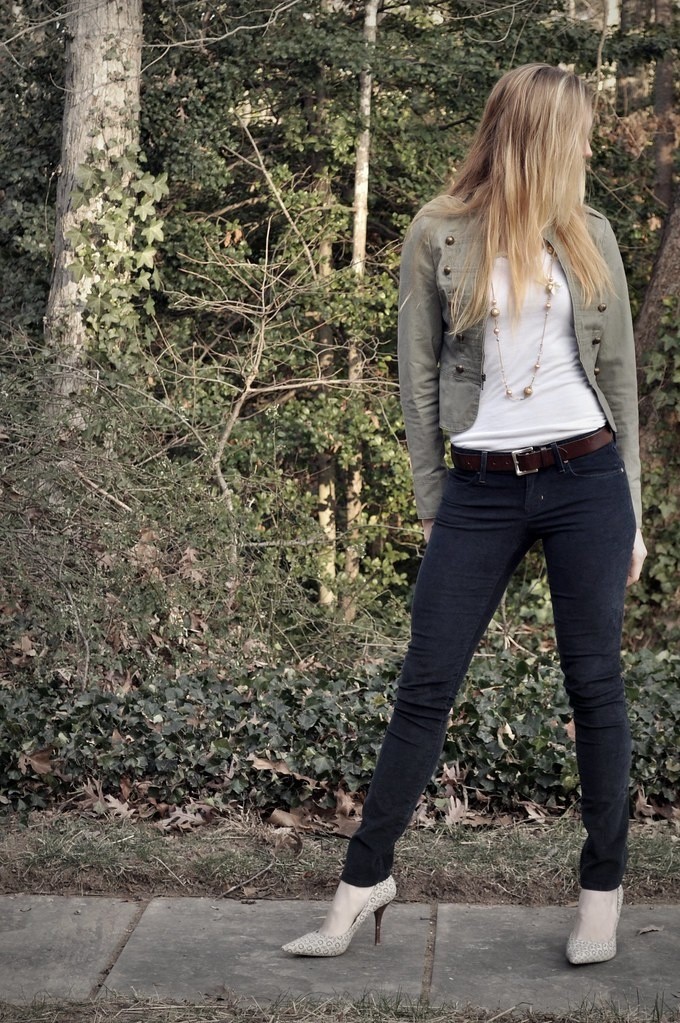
[451,426,612,477]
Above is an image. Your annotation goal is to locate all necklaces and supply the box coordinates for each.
[491,252,556,401]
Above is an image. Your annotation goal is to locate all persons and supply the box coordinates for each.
[282,62,648,965]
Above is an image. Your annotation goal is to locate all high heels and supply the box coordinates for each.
[281,874,396,957]
[566,883,624,965]
